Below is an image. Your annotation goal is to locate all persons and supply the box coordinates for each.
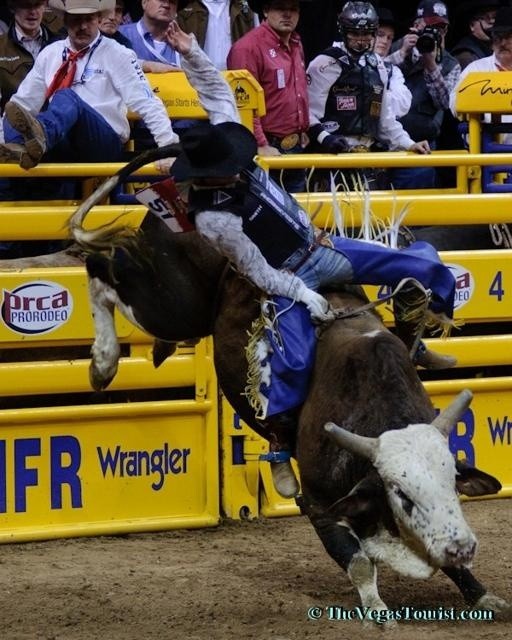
[0,1,512,502]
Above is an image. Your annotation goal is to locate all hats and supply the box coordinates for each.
[480,9,512,37]
[169,121,257,180]
[49,1,116,14]
[417,1,450,26]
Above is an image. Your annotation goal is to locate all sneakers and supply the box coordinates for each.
[5,101,47,159]
[0,144,37,169]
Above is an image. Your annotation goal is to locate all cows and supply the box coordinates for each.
[60,142,512,634]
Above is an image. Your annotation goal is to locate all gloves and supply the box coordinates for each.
[317,130,348,152]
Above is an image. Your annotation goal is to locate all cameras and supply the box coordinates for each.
[415,27,442,55]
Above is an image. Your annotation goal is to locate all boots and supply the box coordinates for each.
[270,412,299,497]
[393,289,455,369]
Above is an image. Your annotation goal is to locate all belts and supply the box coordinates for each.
[293,230,328,273]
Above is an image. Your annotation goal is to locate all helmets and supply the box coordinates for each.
[338,2,379,60]
